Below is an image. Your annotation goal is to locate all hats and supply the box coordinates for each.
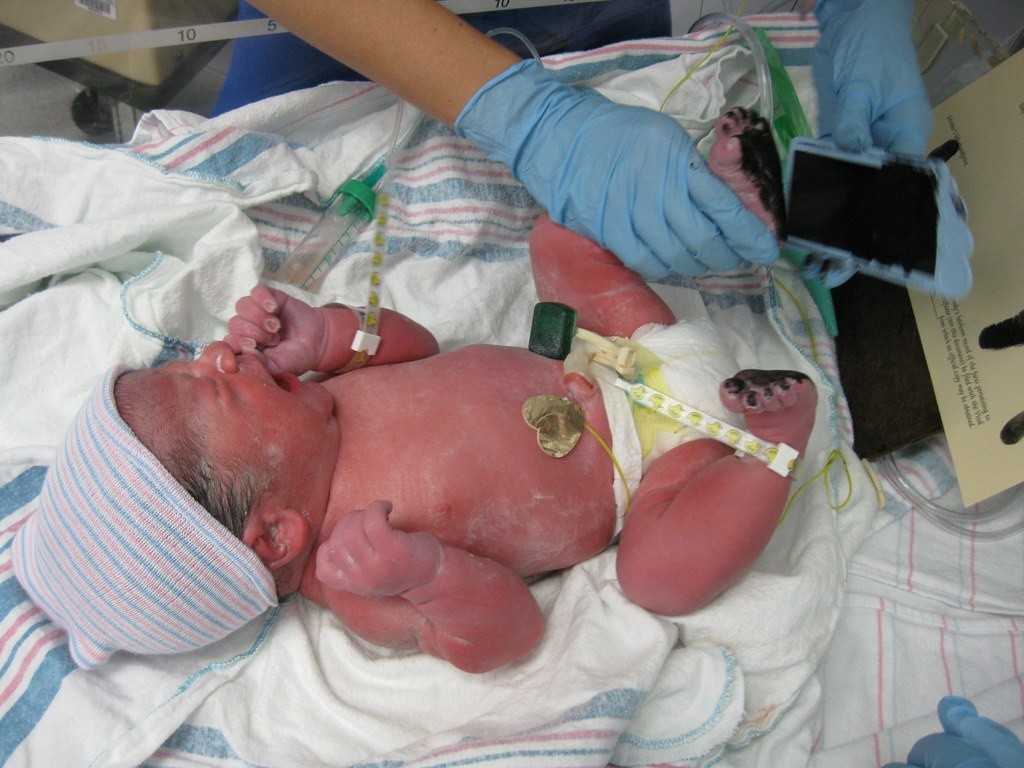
[12,363,279,670]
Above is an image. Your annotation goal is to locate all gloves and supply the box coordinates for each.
[453,58,780,281]
[882,694,1024,768]
[811,0,932,290]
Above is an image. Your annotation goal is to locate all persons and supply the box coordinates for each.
[12,106,819,673]
[213,0,941,288]
